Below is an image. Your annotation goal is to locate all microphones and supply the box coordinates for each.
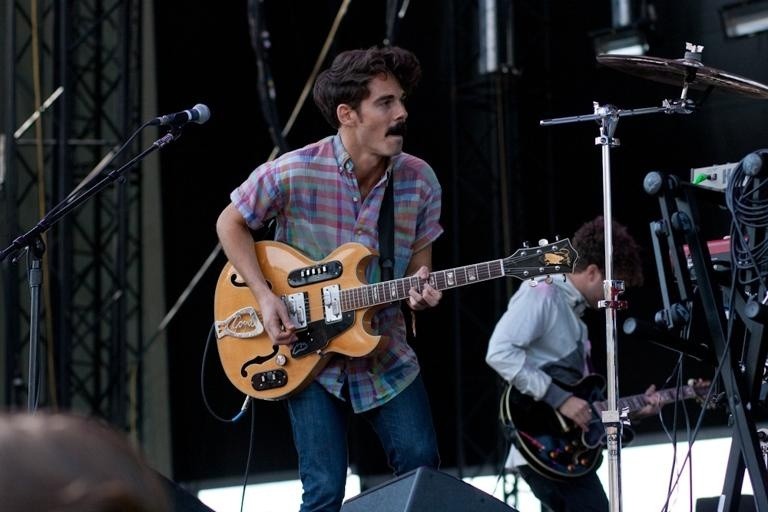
[151,126,182,148]
[146,102,212,125]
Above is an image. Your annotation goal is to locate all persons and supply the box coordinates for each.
[485,217,643,512]
[216,47,444,512]
[0,410,172,512]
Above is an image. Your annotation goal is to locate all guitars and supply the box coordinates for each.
[214,235,581,403]
[499,373,718,483]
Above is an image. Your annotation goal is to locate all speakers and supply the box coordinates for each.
[338,465,520,512]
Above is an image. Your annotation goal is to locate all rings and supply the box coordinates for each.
[588,409,592,414]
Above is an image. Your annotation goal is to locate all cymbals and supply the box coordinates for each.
[595,51,767,101]
